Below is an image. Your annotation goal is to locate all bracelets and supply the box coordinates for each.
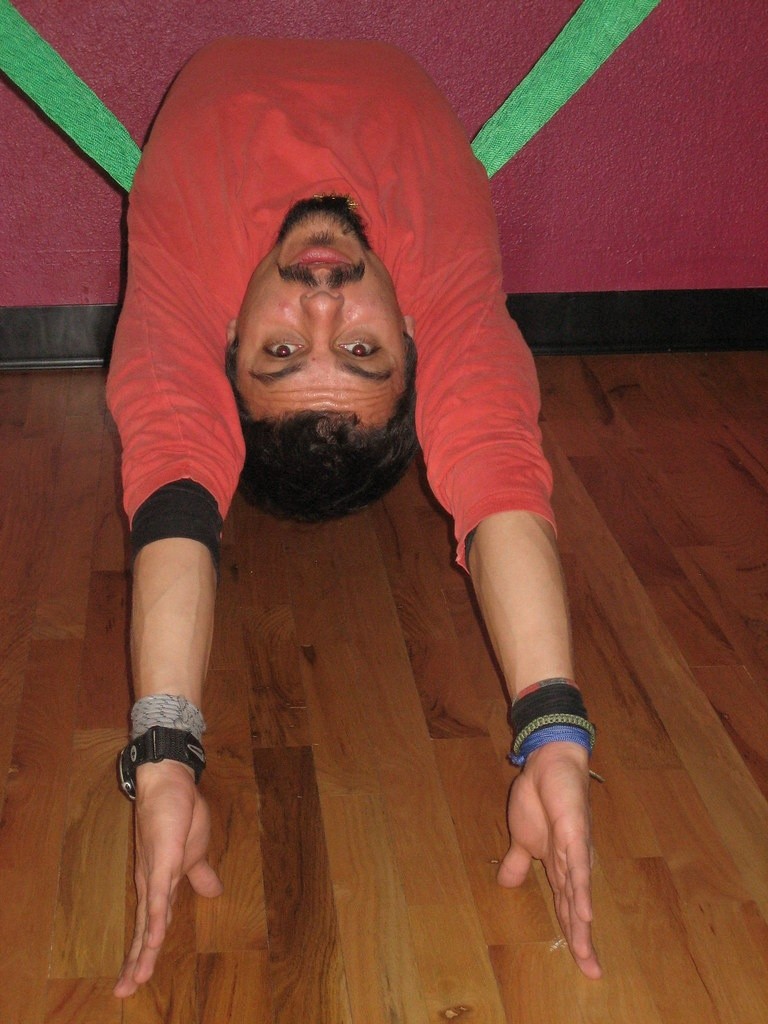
[506,678,595,763]
[129,697,205,744]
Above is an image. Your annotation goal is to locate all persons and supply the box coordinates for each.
[103,30,605,997]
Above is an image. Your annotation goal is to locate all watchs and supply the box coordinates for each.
[115,725,208,799]
